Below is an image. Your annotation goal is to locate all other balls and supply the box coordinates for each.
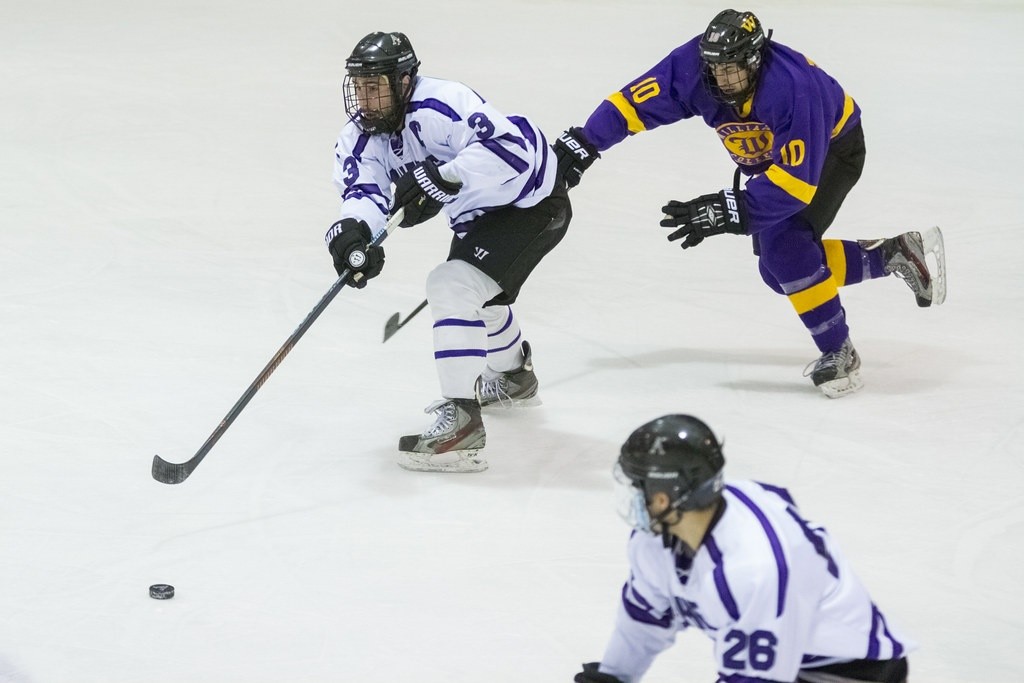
[149,583,176,600]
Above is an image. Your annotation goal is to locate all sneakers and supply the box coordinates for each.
[803,334,865,400]
[858,225,946,308]
[396,398,489,473]
[474,340,543,409]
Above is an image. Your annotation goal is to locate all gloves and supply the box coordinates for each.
[574,662,623,683]
[660,189,748,249]
[386,159,463,228]
[325,218,385,289]
[549,126,601,192]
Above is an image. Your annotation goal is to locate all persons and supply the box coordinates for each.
[326,31,572,472]
[553,9,946,398]
[572,414,909,683]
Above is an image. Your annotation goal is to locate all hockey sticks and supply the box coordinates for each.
[382,300,431,344]
[152,204,405,487]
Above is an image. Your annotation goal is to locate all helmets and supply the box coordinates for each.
[618,415,724,491]
[698,9,765,107]
[345,31,422,134]
